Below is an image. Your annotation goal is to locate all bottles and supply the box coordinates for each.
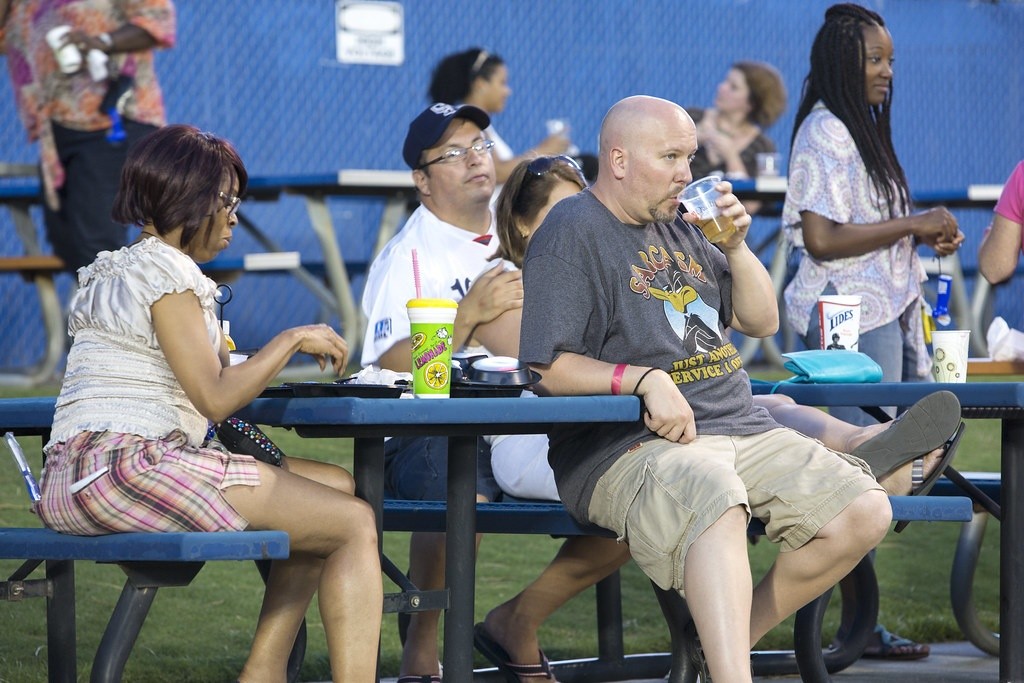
[217,320,236,351]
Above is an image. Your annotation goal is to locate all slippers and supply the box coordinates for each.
[830,623,931,659]
[397,660,441,683]
[474,622,557,683]
[848,390,961,479]
[893,421,966,532]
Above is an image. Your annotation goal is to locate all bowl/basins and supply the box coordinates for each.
[462,355,542,385]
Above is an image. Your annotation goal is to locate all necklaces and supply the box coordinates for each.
[142,231,156,237]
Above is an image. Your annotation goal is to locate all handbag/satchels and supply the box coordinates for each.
[771,349,882,394]
[218,416,283,466]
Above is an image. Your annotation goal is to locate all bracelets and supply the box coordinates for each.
[612,364,629,394]
[98,32,111,46]
[632,367,660,394]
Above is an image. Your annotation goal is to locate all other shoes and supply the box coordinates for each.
[687,619,711,683]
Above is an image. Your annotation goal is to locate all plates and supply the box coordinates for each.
[450,382,523,398]
[257,382,402,398]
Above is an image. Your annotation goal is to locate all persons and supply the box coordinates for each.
[977,161,1024,285]
[521,96,893,683]
[431,48,570,182]
[779,4,966,657]
[0,0,176,272]
[470,159,966,534]
[39,125,384,682]
[359,101,632,683]
[685,61,786,178]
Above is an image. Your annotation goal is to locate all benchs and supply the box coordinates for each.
[0,248,1024,395]
[0,471,1000,683]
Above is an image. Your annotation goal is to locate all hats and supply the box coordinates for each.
[403,103,490,169]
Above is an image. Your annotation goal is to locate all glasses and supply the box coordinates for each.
[418,139,495,169]
[217,189,241,220]
[527,155,590,187]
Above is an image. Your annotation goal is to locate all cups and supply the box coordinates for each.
[756,154,782,176]
[930,330,971,383]
[677,176,735,243]
[229,354,249,365]
[547,118,568,135]
[817,294,862,352]
[406,298,458,398]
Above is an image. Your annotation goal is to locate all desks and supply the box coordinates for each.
[725,175,1003,372]
[0,393,641,683]
[0,168,422,380]
[751,377,1024,683]
